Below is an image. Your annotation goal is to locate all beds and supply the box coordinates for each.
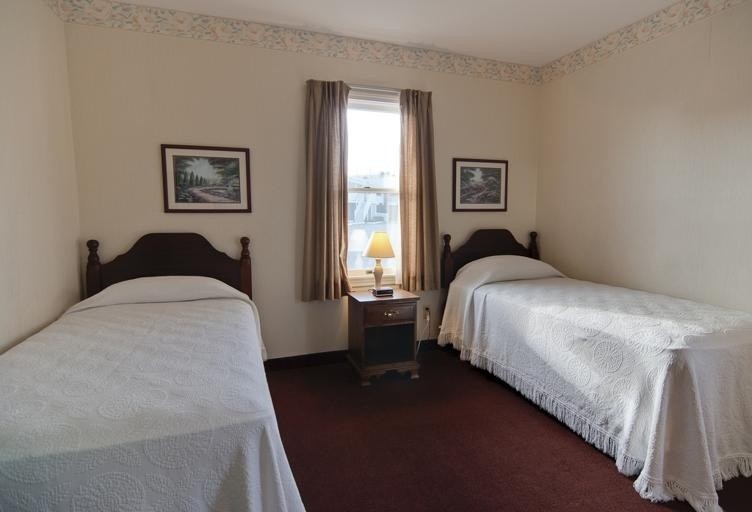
[0,233,311,512]
[442,228,752,502]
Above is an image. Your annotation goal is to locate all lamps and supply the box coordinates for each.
[362,231,396,291]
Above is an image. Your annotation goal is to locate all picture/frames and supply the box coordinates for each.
[450,159,507,211]
[161,143,251,215]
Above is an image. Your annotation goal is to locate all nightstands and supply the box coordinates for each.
[344,288,421,386]
